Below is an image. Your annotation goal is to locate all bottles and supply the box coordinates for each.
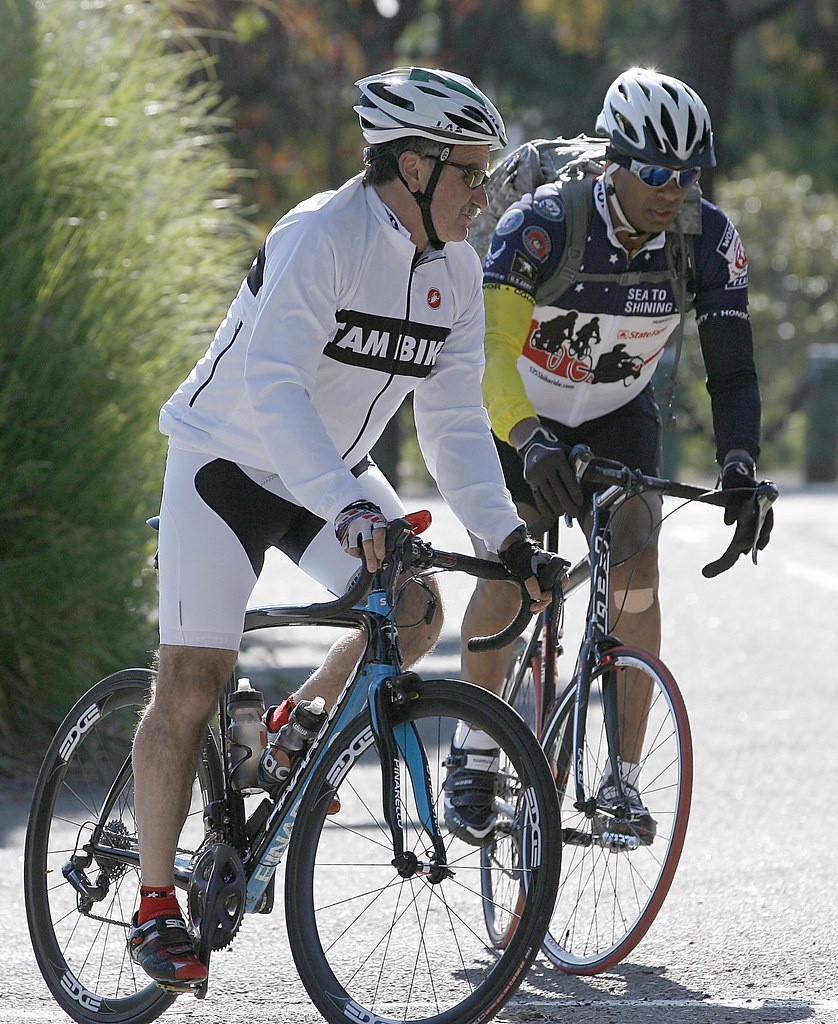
[227,677,269,795]
[257,695,331,789]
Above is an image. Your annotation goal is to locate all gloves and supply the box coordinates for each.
[720,459,774,556]
[517,427,585,522]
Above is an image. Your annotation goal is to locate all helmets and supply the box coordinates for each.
[354,65,509,150]
[596,66,718,168]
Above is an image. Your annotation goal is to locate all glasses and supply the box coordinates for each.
[604,145,702,190]
[422,155,492,189]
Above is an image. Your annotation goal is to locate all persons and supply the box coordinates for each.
[446,63,777,844]
[121,67,568,995]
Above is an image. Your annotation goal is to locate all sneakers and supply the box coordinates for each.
[592,775,658,846]
[127,910,209,985]
[260,705,340,815]
[445,727,499,845]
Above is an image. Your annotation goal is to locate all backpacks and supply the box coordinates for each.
[462,133,701,314]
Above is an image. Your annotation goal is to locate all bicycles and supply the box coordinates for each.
[476,445,780,976]
[23,510,561,1024]
